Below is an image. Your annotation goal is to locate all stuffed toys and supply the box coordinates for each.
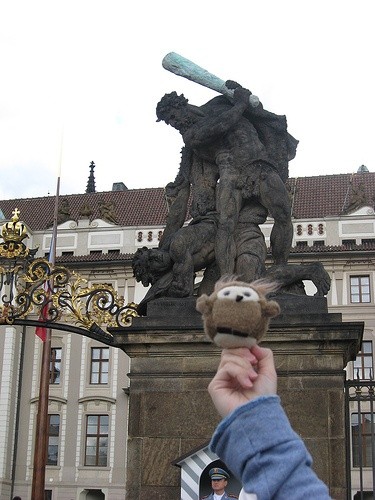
[195,273,280,349]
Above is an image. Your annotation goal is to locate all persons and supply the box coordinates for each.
[201,468,239,500]
[154,78,298,290]
[133,212,331,317]
[205,344,332,500]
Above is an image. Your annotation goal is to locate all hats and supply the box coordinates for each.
[209,468,230,480]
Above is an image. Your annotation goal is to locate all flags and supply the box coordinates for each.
[34,234,53,343]
